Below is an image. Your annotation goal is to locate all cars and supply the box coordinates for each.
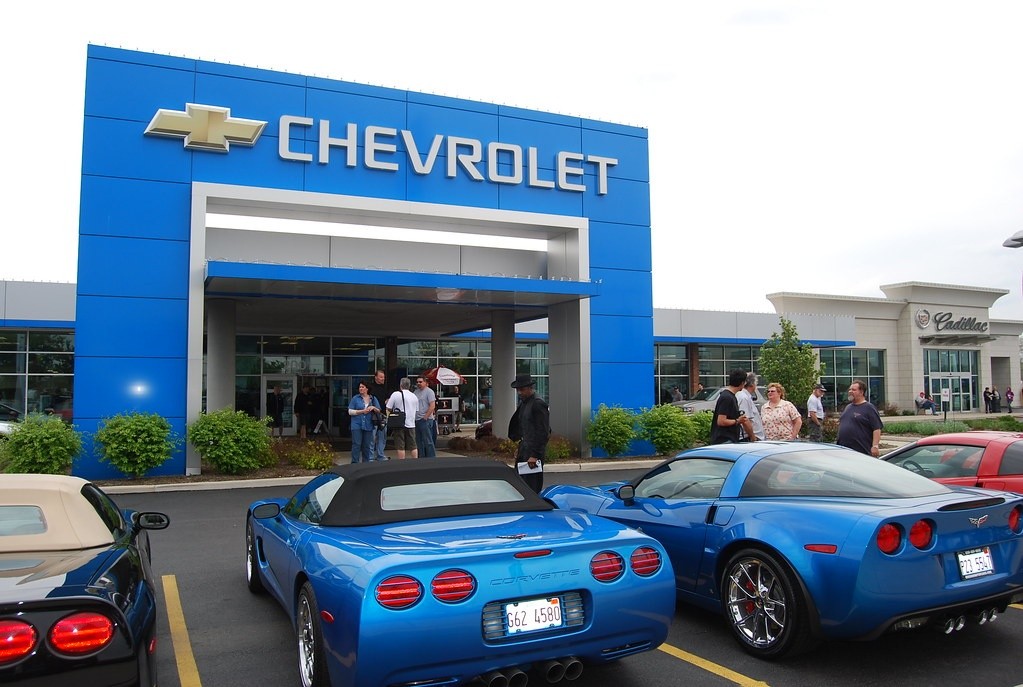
[43,394,74,424]
[941,448,983,469]
[0,404,27,441]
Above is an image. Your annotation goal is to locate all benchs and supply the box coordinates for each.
[915,400,940,415]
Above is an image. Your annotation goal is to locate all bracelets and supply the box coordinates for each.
[874,445,879,448]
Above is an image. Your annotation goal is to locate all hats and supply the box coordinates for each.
[815,384,827,391]
[920,392,925,395]
[511,373,536,388]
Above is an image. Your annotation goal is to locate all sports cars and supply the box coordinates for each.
[0,473,171,687]
[778,430,1022,497]
[244,457,678,687]
[541,440,1023,657]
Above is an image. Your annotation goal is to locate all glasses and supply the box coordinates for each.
[416,381,422,383]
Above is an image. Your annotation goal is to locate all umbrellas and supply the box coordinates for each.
[420,365,468,396]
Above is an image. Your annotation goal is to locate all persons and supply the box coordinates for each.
[917,392,940,416]
[696,383,705,392]
[807,384,827,442]
[836,379,884,457]
[672,386,683,402]
[449,386,465,433]
[982,386,1003,412]
[267,385,285,441]
[761,383,803,441]
[709,369,747,446]
[735,372,765,442]
[297,383,329,440]
[1005,386,1014,413]
[508,373,551,493]
[349,370,440,464]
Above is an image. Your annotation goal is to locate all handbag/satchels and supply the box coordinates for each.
[388,408,406,430]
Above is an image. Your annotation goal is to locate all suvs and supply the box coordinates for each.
[665,385,769,415]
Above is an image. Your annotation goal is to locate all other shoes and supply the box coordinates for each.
[452,427,462,433]
